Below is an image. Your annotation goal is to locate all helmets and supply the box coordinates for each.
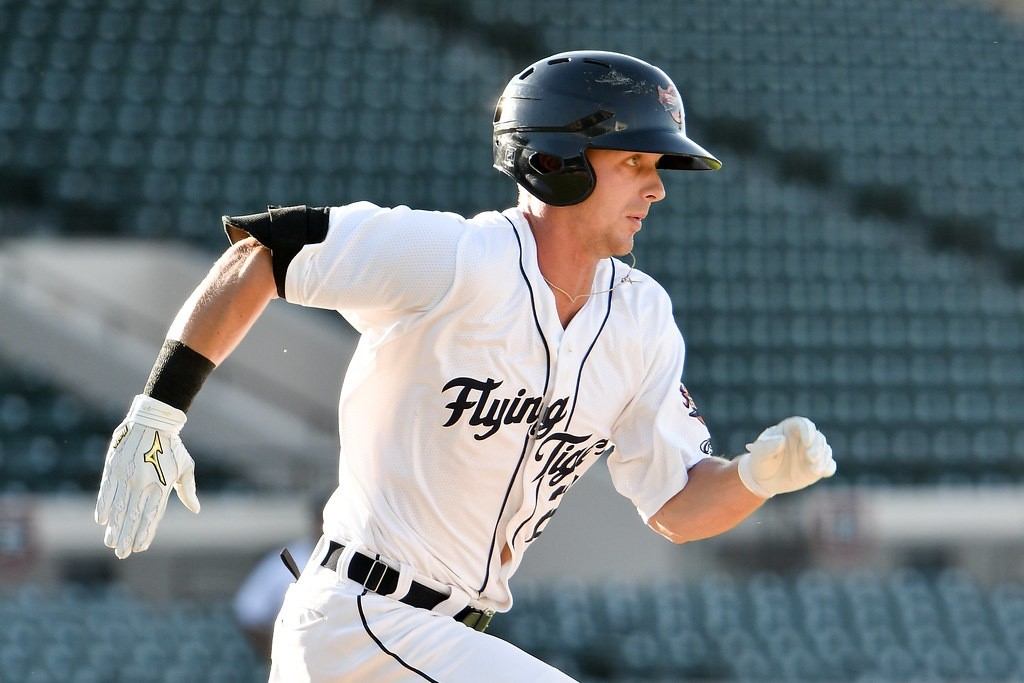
[493,51,722,207]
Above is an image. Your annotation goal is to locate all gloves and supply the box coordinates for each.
[96,395,201,558]
[738,414,837,500]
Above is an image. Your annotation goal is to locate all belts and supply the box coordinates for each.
[322,537,493,632]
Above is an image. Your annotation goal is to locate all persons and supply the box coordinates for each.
[94,49,837,683]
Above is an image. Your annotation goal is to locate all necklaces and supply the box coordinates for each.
[541,251,635,302]
